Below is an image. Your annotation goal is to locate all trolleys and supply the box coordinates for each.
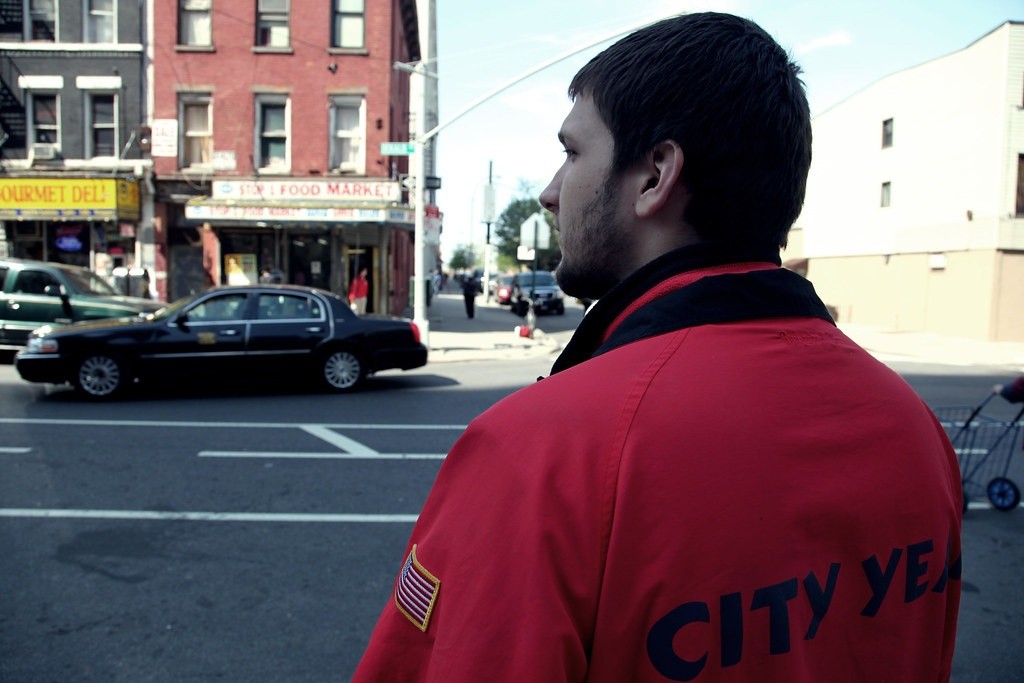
[931,388,1024,515]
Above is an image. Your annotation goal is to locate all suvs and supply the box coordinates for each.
[510,273,564,317]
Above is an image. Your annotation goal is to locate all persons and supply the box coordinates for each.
[462,277,477,319]
[347,10,965,683]
[348,267,369,315]
[993,376,1024,403]
[259,267,279,283]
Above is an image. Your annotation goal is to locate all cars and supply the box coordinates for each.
[0,255,197,352]
[12,283,427,402]
[454,268,511,304]
